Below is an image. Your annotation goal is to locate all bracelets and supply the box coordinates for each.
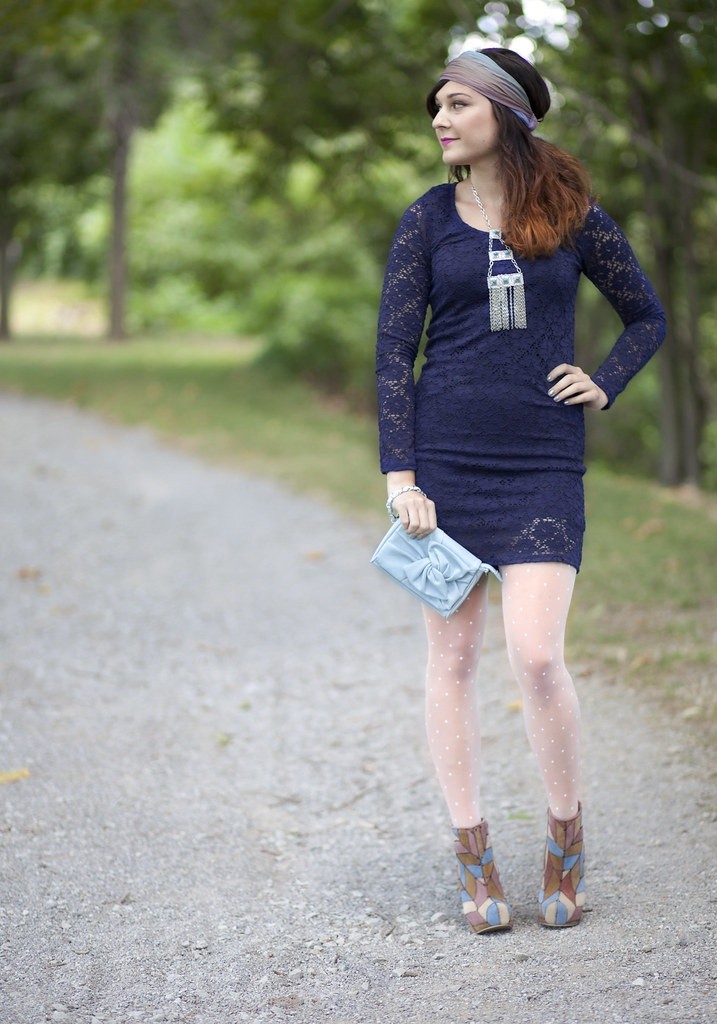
[386,485,427,523]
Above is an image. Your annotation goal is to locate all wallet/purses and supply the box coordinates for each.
[370,517,503,619]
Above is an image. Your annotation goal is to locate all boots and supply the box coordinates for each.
[536,801,585,926]
[451,818,513,934]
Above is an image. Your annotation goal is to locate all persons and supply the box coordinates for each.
[375,48,666,934]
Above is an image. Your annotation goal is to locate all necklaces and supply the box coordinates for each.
[469,180,528,332]
